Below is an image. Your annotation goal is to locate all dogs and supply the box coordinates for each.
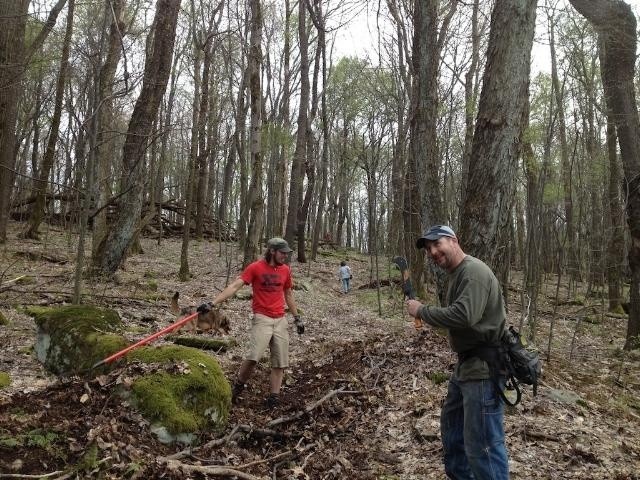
[164,292,231,341]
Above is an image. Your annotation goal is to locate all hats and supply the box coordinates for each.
[268,237,293,253]
[416,225,457,250]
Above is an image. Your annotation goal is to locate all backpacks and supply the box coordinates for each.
[501,324,542,385]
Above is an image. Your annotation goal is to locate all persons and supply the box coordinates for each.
[339,260,354,295]
[196,236,306,408]
[407,225,512,480]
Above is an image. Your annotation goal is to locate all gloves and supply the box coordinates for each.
[350,275,353,279]
[294,315,305,334]
[197,301,215,315]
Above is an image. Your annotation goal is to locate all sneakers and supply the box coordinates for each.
[232,382,245,400]
[267,393,282,413]
[345,288,350,294]
